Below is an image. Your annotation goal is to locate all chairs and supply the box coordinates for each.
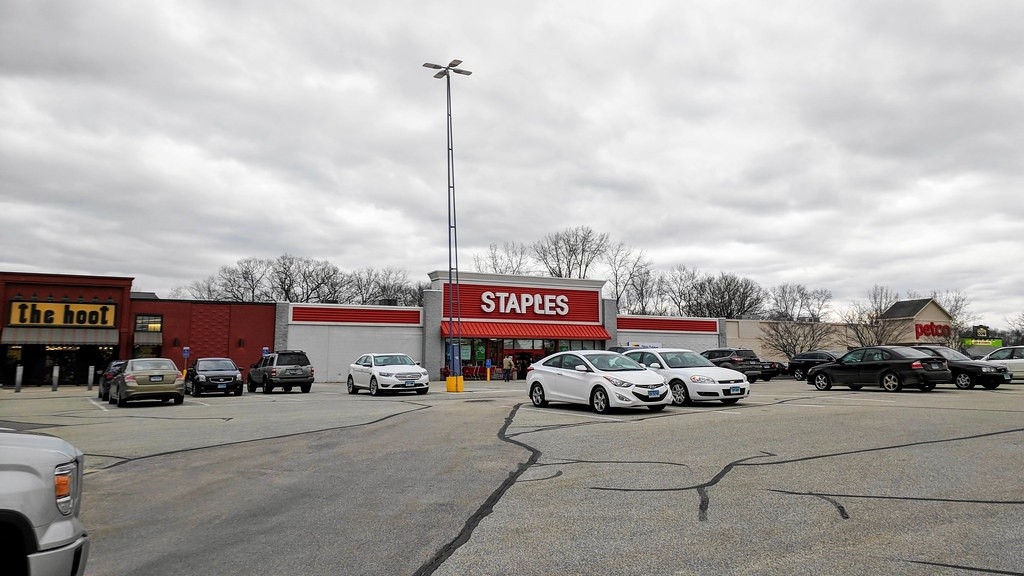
[596,357,611,367]
[668,357,681,365]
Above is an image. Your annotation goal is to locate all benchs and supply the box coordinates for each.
[552,359,582,369]
[134,364,170,370]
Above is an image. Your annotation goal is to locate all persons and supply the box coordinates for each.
[503,355,514,382]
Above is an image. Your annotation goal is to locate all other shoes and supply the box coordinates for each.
[505,378,507,382]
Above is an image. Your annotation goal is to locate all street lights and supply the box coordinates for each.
[423,59,472,393]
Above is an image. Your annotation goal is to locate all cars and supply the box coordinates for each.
[347,353,429,396]
[910,345,1013,389]
[758,356,787,381]
[806,346,952,393]
[788,350,860,381]
[622,348,751,407]
[526,350,672,415]
[108,358,184,408]
[98,361,125,401]
[184,358,244,397]
[980,346,1024,384]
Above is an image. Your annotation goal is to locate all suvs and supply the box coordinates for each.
[700,345,762,384]
[247,350,314,394]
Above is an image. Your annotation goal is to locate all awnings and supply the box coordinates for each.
[133,332,162,346]
[1,327,119,346]
[441,321,611,340]
